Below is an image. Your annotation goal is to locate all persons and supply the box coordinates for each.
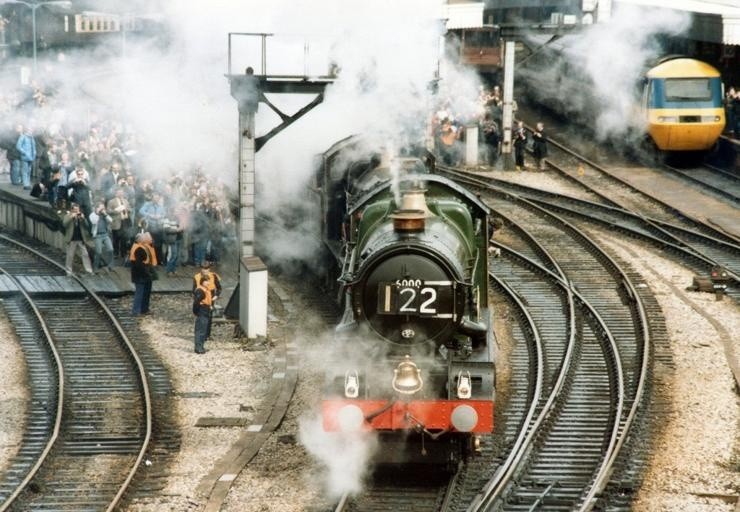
[143,232,159,315]
[1,49,231,278]
[373,66,549,176]
[723,86,740,141]
[191,275,214,354]
[128,232,152,314]
[192,262,223,342]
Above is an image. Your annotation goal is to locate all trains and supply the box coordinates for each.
[444,23,504,98]
[306,123,500,491]
[515,23,730,162]
